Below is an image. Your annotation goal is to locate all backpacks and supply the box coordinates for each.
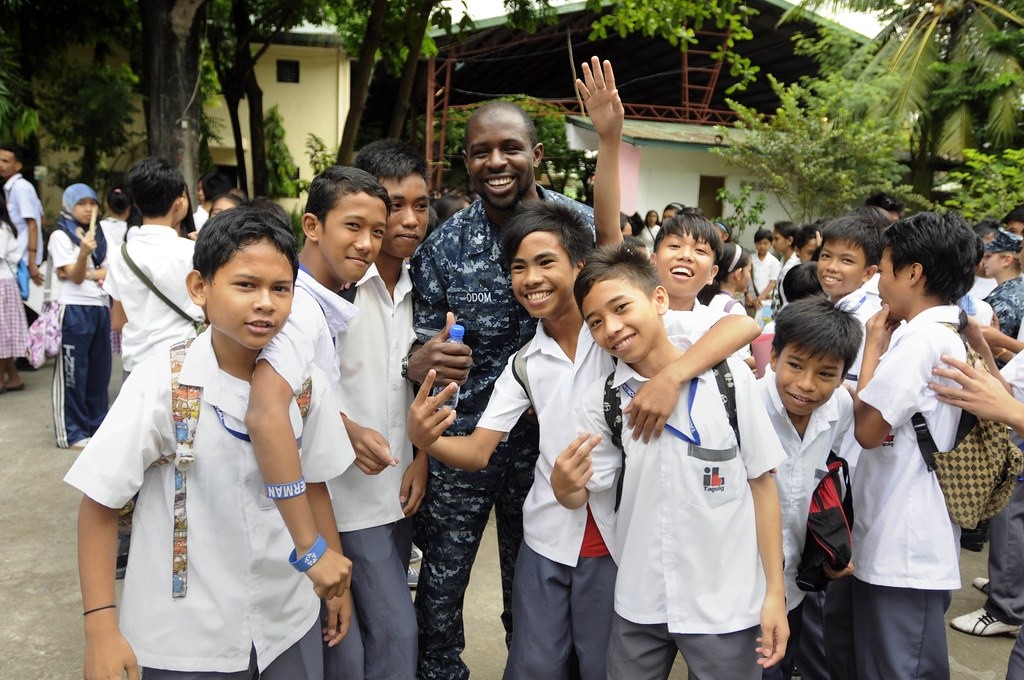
[911,322,1024,530]
[795,450,854,592]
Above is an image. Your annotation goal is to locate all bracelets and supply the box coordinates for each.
[265,477,305,500]
[83,605,116,615]
[996,348,1006,358]
[288,534,327,572]
[29,249,36,252]
[744,291,749,296]
[89,271,93,280]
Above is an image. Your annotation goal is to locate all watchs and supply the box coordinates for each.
[401,352,413,383]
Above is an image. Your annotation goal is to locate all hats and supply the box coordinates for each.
[983,226,1023,254]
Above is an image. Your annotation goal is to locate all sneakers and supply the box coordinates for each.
[949,608,1022,638]
[973,577,989,590]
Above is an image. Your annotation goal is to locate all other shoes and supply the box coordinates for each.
[72,437,91,448]
[0,380,24,394]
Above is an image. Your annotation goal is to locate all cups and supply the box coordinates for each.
[751,333,775,378]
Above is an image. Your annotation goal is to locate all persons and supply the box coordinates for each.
[0,55,1024,680]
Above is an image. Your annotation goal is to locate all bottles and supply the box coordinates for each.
[431,325,467,410]
